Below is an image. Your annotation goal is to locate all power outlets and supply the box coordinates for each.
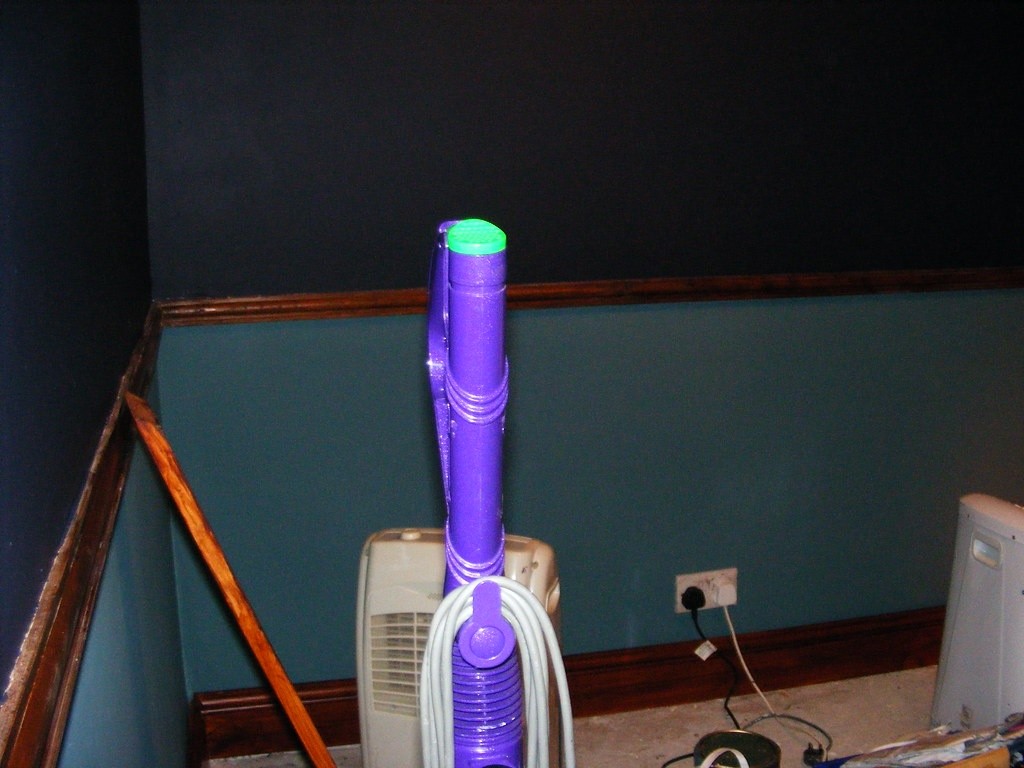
[675,568,737,613]
